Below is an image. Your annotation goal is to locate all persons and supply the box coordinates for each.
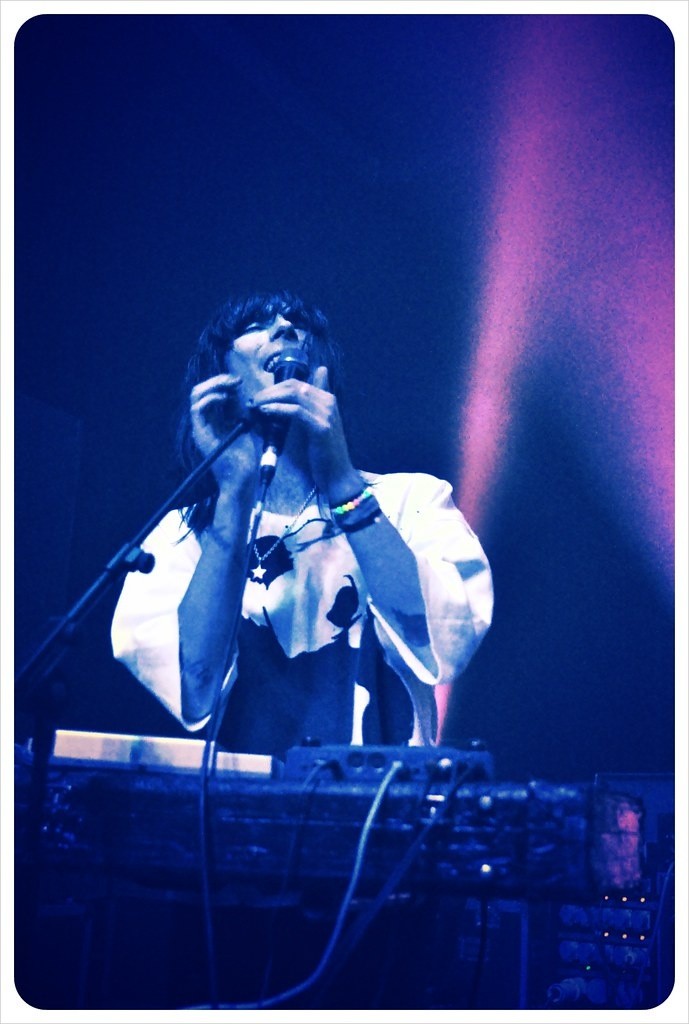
[109,288,494,760]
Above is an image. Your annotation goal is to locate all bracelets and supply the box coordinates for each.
[330,484,382,533]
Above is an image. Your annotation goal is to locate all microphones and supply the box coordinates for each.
[259,348,309,482]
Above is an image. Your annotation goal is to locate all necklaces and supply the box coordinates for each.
[248,485,318,580]
